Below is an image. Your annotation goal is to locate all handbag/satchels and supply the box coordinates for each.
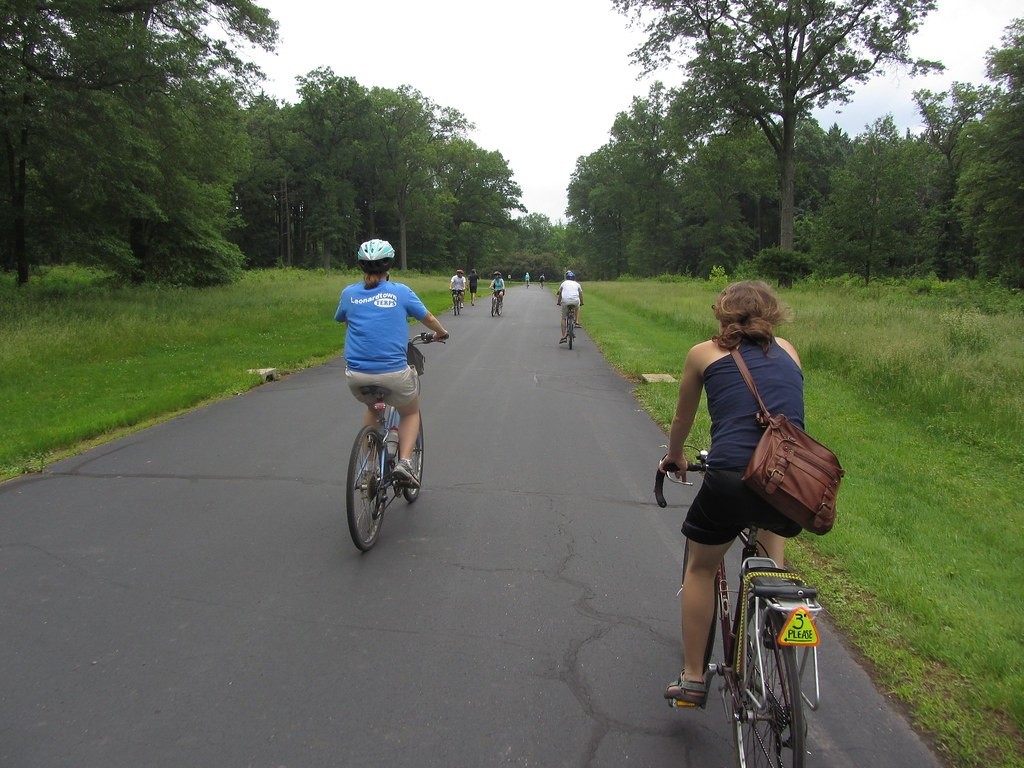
[742,413,845,536]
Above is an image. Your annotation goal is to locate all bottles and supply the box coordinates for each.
[386,426,399,458]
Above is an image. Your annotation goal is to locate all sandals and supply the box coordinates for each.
[763,624,782,650]
[663,670,707,705]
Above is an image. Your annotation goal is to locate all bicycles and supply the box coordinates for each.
[539,281,544,289]
[556,303,584,351]
[449,289,465,316]
[654,445,824,768]
[491,288,504,317]
[526,279,529,288]
[347,328,450,553]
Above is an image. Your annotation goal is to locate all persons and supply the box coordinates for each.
[334,238,447,489]
[525,272,529,285]
[659,279,804,706]
[468,269,479,306]
[490,271,506,313]
[557,270,583,340]
[540,274,545,286]
[449,269,466,310]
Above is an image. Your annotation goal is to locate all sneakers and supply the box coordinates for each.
[361,468,381,488]
[392,459,420,488]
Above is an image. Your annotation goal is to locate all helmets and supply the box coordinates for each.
[358,238,394,262]
[493,271,502,276]
[456,269,464,274]
[565,271,575,280]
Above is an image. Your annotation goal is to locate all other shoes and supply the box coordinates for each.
[452,306,454,309]
[559,337,567,344]
[461,303,463,308]
[574,323,581,328]
